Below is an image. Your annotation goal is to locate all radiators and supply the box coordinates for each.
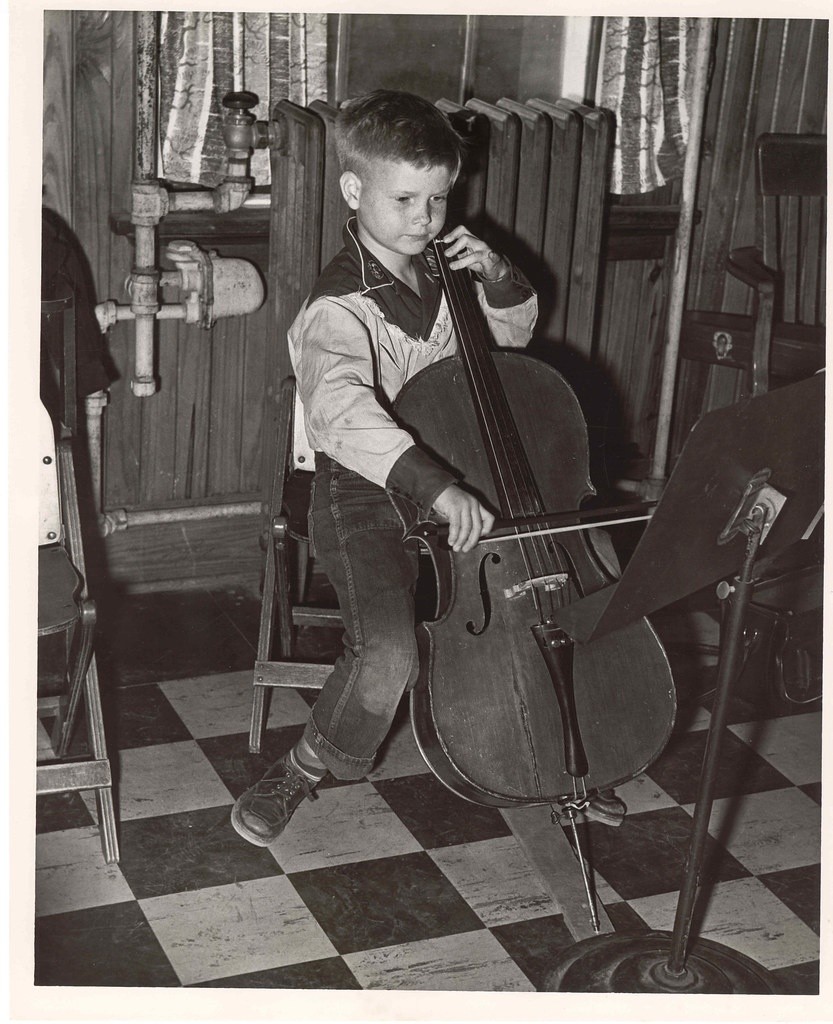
[223,93,609,555]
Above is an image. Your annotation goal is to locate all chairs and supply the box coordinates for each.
[724,132,828,403]
[36,399,121,865]
[249,378,432,753]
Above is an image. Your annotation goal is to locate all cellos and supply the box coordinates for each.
[380,233,682,935]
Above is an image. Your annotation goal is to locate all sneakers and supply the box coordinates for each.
[231,753,317,848]
[581,789,626,827]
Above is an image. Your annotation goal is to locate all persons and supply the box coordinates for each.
[231,88,628,849]
